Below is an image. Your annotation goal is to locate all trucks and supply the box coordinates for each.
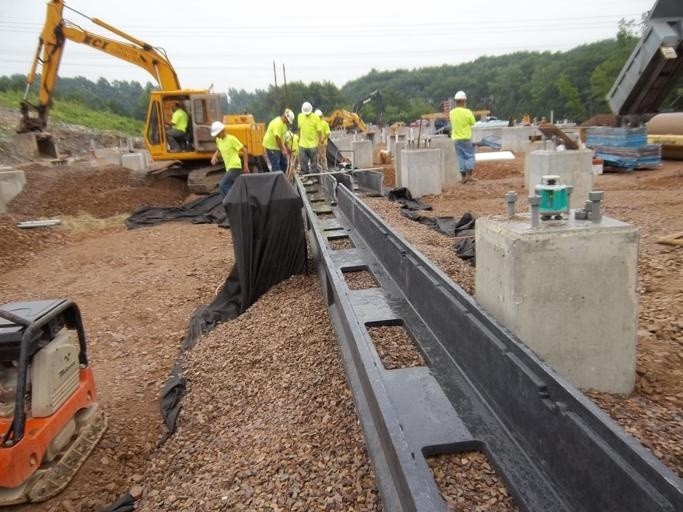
[605,0,683,129]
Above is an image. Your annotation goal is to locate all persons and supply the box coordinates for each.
[448,88,477,185]
[285,130,299,160]
[313,107,330,174]
[260,108,296,174]
[296,101,324,184]
[208,120,250,201]
[162,102,189,154]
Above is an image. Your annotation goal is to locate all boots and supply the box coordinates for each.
[460,170,478,184]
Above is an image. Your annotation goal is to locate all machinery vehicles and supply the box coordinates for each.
[1,299,108,506]
[16,0,265,194]
[323,88,492,140]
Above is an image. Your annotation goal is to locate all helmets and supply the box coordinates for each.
[454,90,468,101]
[210,121,225,137]
[315,110,323,117]
[284,109,294,125]
[301,102,312,115]
